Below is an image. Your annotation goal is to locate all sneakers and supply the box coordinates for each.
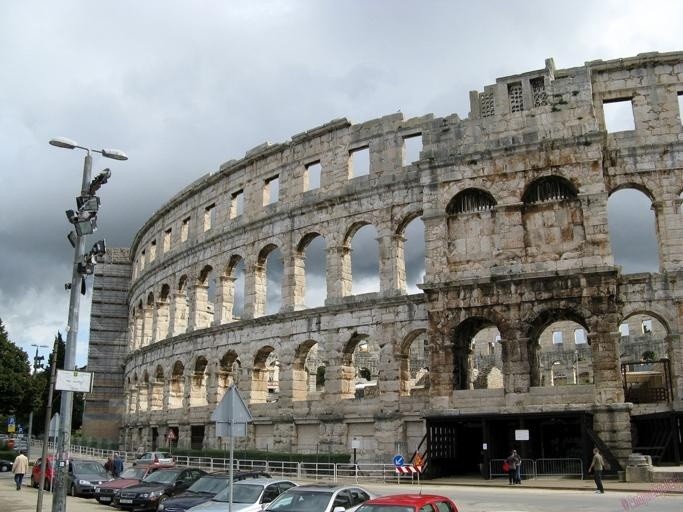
[596,490,604,494]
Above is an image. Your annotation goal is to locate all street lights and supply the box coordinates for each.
[28,344,49,447]
[50,137,127,509]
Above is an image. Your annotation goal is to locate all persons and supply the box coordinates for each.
[587,448,604,495]
[104,455,116,479]
[11,450,28,492]
[478,450,483,476]
[512,449,521,485]
[503,452,519,486]
[113,452,123,478]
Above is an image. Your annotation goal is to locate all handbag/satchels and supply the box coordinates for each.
[502,463,510,471]
[602,464,610,471]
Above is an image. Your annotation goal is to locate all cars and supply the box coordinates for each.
[95,452,302,512]
[31,456,114,496]
[1,438,27,472]
[264,484,457,512]
